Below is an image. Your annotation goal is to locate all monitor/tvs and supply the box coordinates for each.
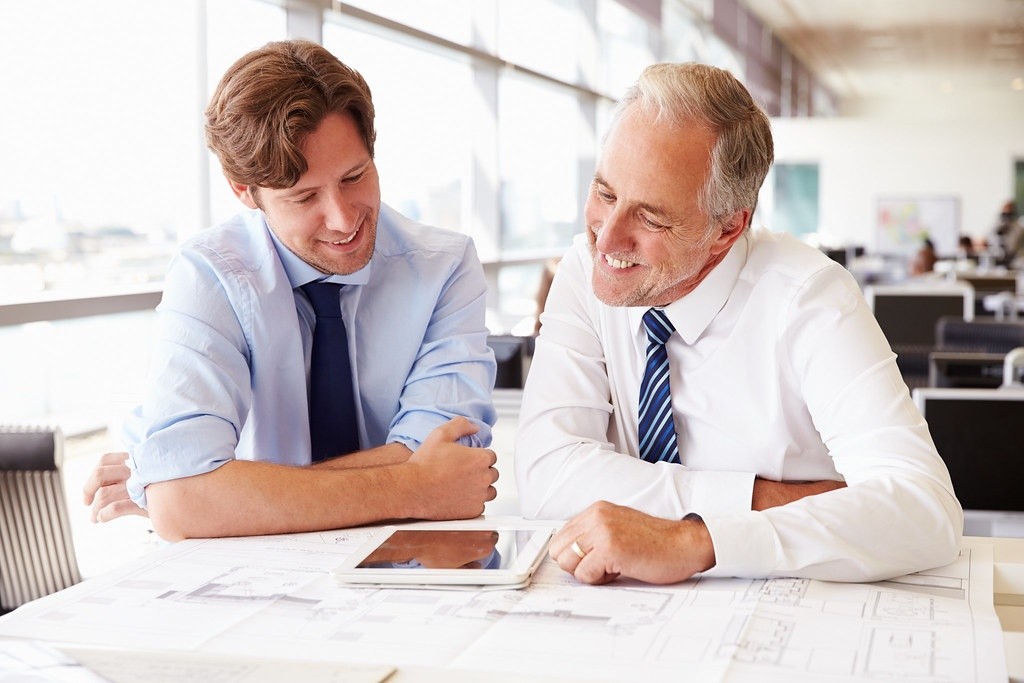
[928,353,1010,389]
[865,282,974,347]
[914,387,1024,540]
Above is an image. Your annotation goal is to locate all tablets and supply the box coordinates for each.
[343,525,557,585]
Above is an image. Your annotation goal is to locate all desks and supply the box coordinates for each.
[0,507,1024,683]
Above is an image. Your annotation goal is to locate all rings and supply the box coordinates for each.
[571,539,585,559]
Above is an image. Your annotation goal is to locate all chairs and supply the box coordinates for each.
[864,285,1024,514]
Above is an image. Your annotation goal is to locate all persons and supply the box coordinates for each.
[822,199,1024,285]
[81,39,499,541]
[513,61,964,585]
[362,530,502,570]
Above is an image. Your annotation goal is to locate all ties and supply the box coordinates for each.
[638,308,682,464]
[299,282,361,466]
